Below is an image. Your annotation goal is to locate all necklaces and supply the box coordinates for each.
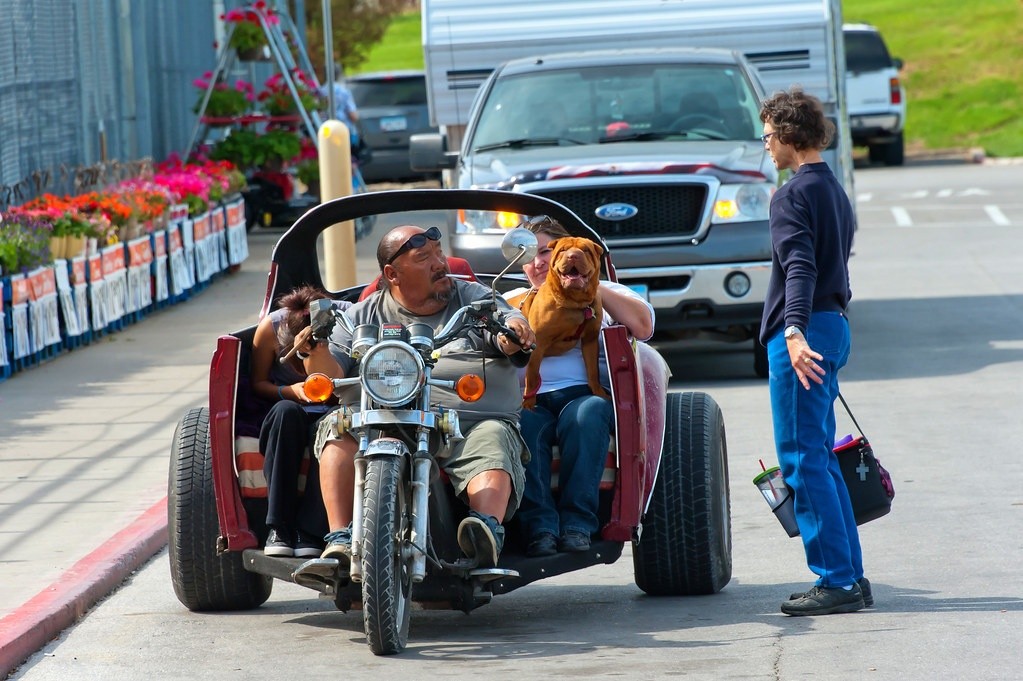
[798,162,811,168]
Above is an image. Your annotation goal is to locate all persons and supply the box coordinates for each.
[253,286,354,556]
[501,216,654,557]
[650,90,742,135]
[759,85,874,617]
[294,225,536,571]
[319,63,365,231]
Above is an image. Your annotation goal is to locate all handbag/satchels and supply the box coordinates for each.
[764,434,894,538]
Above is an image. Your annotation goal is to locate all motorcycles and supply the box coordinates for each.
[164,190,732,657]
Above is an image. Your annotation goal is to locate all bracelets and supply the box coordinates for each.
[277,385,285,400]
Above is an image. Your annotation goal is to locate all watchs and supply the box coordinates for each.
[784,326,804,339]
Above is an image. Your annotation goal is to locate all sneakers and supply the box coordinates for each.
[264,528,324,558]
[529,530,592,556]
[455,512,505,568]
[321,533,352,560]
[782,579,876,616]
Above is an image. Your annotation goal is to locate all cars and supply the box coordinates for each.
[406,50,782,380]
[338,72,439,185]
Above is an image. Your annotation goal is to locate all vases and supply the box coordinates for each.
[67,234,83,258]
[179,202,190,222]
[126,219,138,239]
[166,204,179,224]
[88,237,98,255]
[118,225,127,241]
[138,224,145,235]
[48,237,59,257]
[237,42,270,62]
[58,237,67,258]
[80,235,89,256]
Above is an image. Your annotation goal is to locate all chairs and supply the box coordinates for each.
[356,250,502,304]
[523,100,572,140]
[667,91,731,134]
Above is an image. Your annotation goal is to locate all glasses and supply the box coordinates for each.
[514,213,556,229]
[760,129,784,142]
[382,225,441,265]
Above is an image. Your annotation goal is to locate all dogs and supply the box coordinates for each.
[505,236,612,410]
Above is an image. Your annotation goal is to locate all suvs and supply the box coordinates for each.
[840,19,909,167]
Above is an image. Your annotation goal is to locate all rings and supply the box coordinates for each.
[803,358,811,364]
[528,328,535,332]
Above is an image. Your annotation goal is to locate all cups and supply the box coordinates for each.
[752,468,799,538]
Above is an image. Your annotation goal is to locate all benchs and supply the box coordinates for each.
[239,328,612,510]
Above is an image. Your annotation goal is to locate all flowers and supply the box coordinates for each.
[0,0,330,274]
